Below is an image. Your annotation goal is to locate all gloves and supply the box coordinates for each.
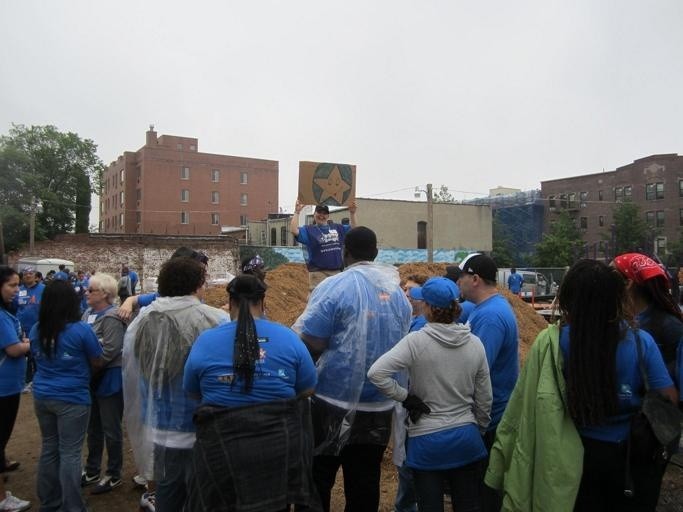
[402,392,431,424]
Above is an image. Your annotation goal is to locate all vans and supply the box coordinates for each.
[520,272,548,297]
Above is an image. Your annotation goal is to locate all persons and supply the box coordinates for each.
[241,255,266,281]
[184,278,317,512]
[368,281,493,511]
[116,267,132,304]
[123,263,138,296]
[33,263,87,302]
[600,253,683,458]
[448,253,518,445]
[488,260,677,512]
[74,271,88,316]
[400,274,436,337]
[509,267,522,299]
[293,228,412,512]
[0,475,31,512]
[119,258,231,512]
[291,202,358,300]
[12,269,44,381]
[1,269,32,473]
[28,280,106,512]
[118,246,209,321]
[81,273,126,495]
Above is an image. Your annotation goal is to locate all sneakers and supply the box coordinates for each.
[133,475,148,491]
[0,491,31,512]
[91,476,123,493]
[80,472,101,486]
[140,491,157,512]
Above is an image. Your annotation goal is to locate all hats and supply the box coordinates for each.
[315,205,329,215]
[447,251,498,283]
[409,277,461,308]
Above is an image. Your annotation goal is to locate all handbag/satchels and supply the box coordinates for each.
[620,320,683,510]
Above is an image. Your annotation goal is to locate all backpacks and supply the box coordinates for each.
[116,276,129,299]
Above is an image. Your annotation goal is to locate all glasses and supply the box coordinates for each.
[86,288,100,293]
